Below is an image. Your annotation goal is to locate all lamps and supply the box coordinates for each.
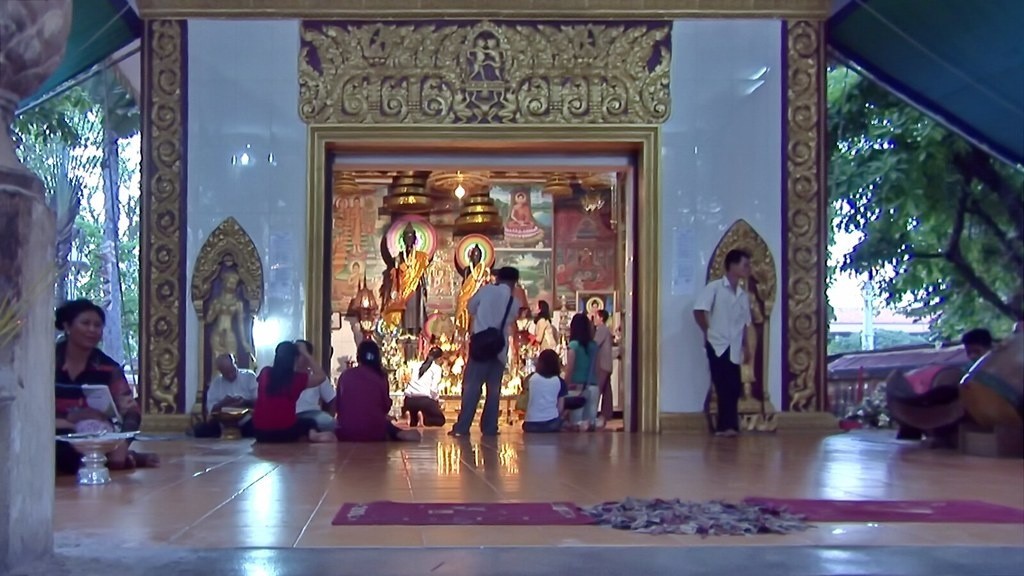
[427,169,489,199]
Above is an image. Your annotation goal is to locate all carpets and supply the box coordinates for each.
[331,496,1024,541]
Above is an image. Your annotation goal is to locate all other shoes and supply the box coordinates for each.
[715,426,738,438]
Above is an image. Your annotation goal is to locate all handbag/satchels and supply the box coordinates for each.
[515,373,534,411]
[468,327,507,360]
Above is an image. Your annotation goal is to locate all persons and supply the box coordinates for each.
[53,297,160,475]
[454,245,491,335]
[693,249,753,435]
[521,299,614,433]
[449,268,520,435]
[961,328,993,363]
[389,230,429,336]
[192,340,446,443]
